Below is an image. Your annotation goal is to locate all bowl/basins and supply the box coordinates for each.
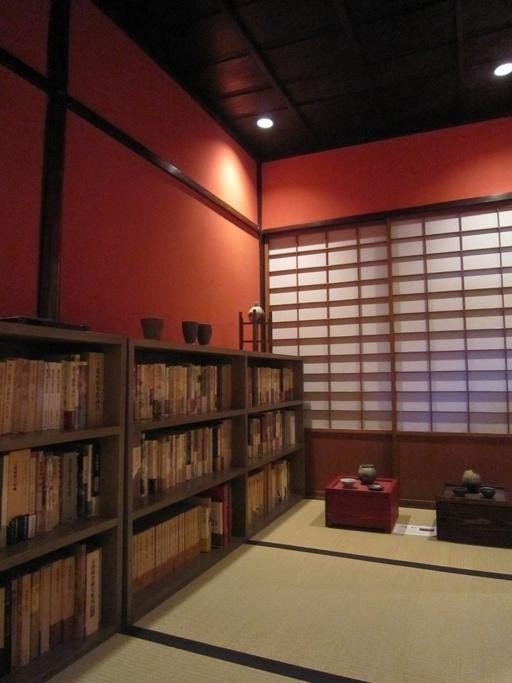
[480,486,498,496]
[452,486,468,494]
[340,478,356,488]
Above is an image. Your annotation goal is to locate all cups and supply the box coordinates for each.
[140,318,163,340]
[182,321,198,344]
[197,321,213,345]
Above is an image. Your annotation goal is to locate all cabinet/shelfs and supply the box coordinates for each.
[124,336,246,628]
[1,322,126,680]
[246,351,307,539]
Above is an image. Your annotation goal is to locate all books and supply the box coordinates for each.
[241,355,299,529]
[1,352,104,674]
[132,363,232,599]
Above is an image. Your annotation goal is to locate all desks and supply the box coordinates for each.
[325,476,398,534]
[435,481,512,548]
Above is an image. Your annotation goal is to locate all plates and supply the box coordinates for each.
[368,482,384,489]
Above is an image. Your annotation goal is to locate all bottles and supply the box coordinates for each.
[248,302,266,323]
[358,464,377,483]
